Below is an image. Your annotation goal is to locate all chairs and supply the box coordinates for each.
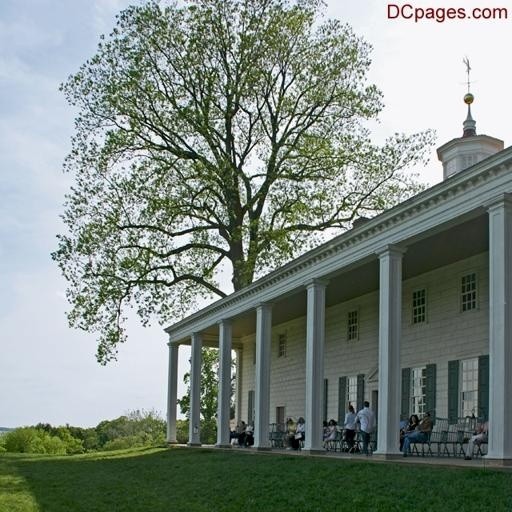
[238,418,487,458]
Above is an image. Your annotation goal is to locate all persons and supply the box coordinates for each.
[285,417,306,452]
[464,416,488,460]
[321,401,376,453]
[400,412,433,455]
[230,421,254,447]
